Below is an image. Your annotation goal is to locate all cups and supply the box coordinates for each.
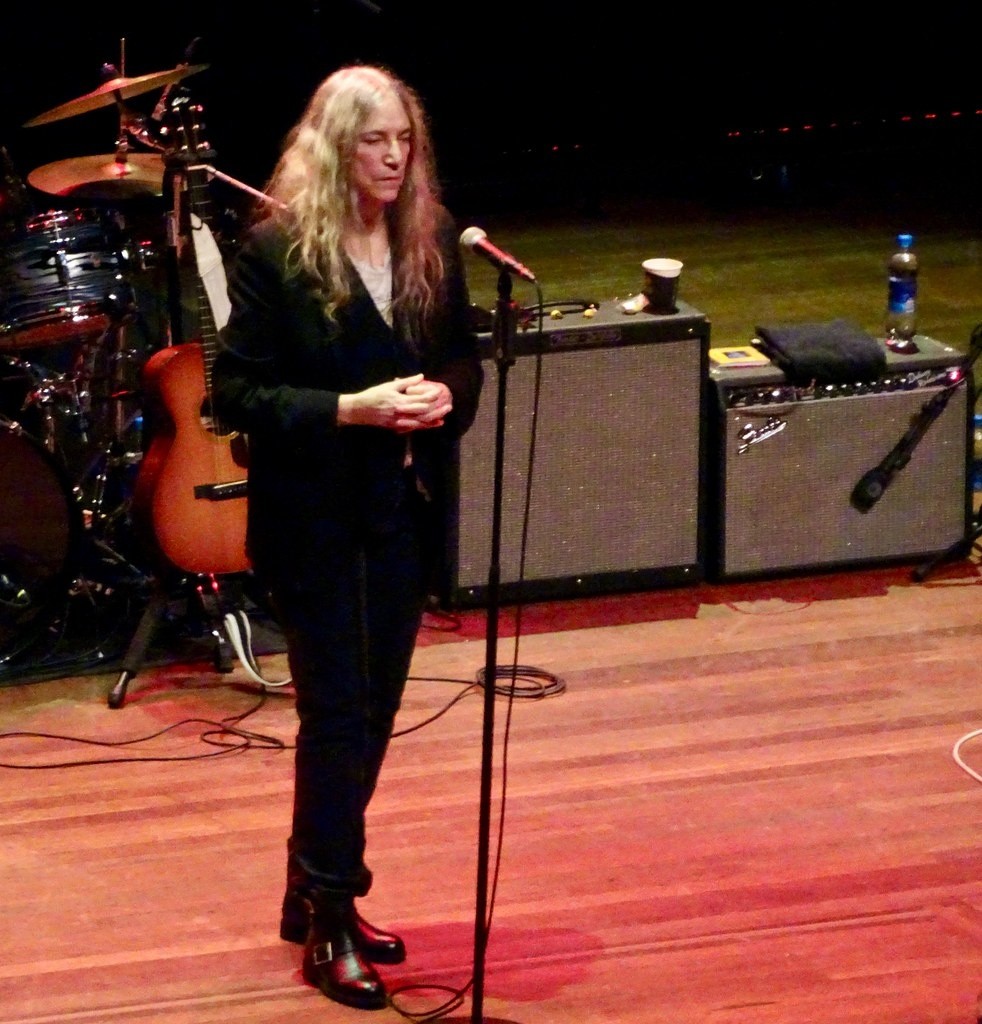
[642,258,682,312]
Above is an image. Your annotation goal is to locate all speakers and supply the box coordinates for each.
[452,290,711,609]
[706,333,977,584]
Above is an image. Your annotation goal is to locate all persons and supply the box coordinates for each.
[211,65,486,1010]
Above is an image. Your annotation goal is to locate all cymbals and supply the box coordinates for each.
[27,152,184,195]
[26,61,209,129]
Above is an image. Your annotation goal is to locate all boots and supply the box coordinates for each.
[300,887,390,1011]
[280,861,406,965]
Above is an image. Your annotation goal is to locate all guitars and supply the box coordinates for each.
[134,98,249,576]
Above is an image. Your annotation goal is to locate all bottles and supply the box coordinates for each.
[885,234,918,349]
[131,417,144,451]
[973,414,982,492]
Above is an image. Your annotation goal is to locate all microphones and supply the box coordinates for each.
[850,394,945,514]
[460,226,537,284]
[152,42,198,121]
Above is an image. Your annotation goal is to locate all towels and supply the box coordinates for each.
[752,320,889,385]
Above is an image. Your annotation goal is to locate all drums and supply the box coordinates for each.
[0,202,141,351]
[1,408,76,628]
